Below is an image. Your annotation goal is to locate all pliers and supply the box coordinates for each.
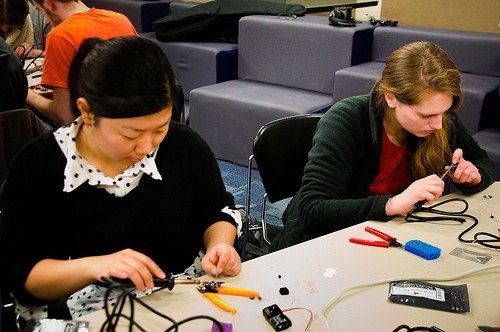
[349,227,404,248]
[196,286,259,314]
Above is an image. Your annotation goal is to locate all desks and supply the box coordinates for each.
[332,24,500,135]
[72,181,500,332]
[80,0,170,33]
[23,57,54,98]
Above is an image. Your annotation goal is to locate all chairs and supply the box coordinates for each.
[188,15,375,170]
[139,2,239,100]
[469,127,500,181]
[0,109,51,186]
[242,113,325,262]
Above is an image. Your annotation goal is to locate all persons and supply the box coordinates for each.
[0,0,56,58]
[268,42,497,254]
[0,35,243,332]
[26,0,140,124]
